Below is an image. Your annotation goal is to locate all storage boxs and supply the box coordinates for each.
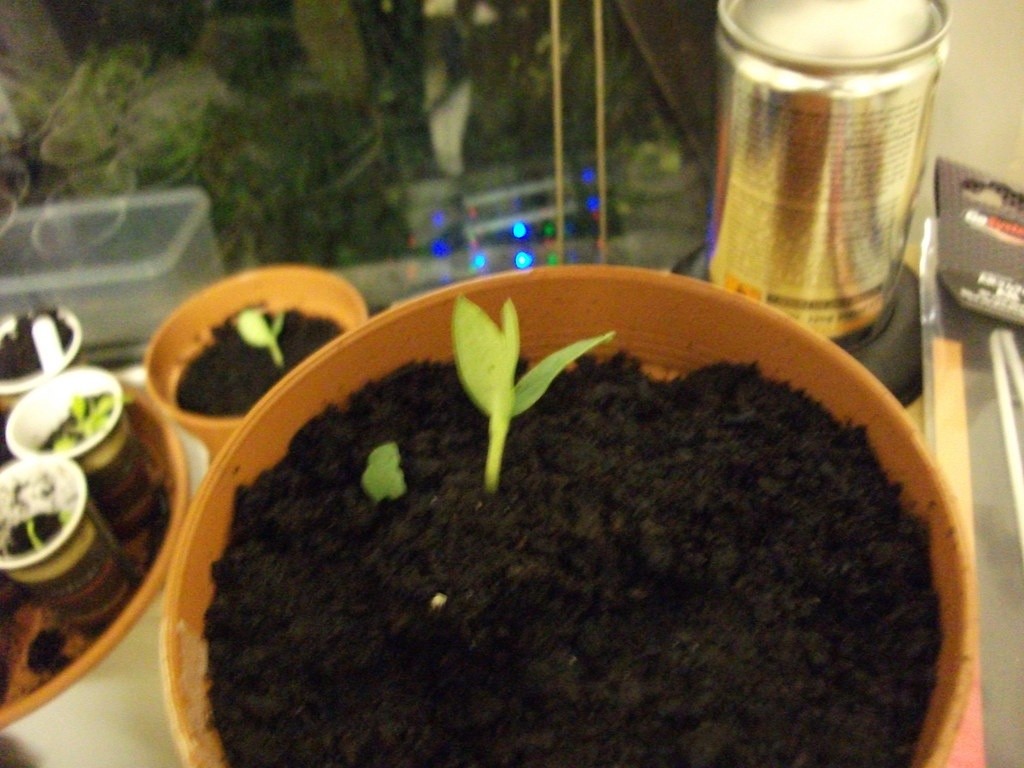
[0,189,226,361]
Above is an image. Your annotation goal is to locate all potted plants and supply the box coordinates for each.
[143,262,370,468]
[161,265,980,768]
[0,306,82,412]
[5,369,158,543]
[0,454,139,636]
